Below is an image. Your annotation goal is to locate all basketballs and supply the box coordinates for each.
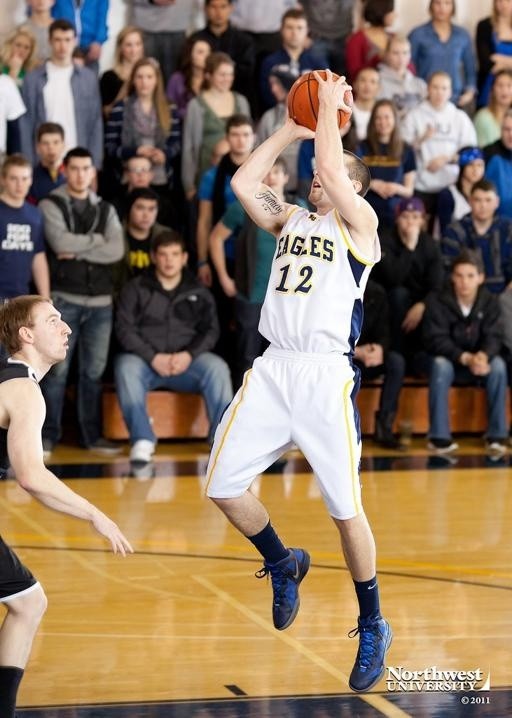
[288,70,352,129]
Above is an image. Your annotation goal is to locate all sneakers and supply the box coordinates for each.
[91,438,124,453]
[348,612,392,693]
[129,439,155,464]
[43,438,52,457]
[264,548,310,630]
[429,438,458,454]
[485,439,507,453]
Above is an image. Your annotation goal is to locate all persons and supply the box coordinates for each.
[0,1,511,467]
[0,295,134,716]
[200,67,395,697]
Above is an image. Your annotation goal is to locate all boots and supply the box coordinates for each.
[373,408,402,447]
